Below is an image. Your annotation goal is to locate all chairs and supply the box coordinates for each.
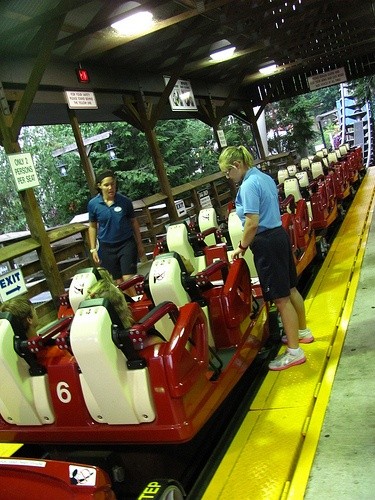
[0,138,362,500]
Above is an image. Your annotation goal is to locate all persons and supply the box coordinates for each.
[88,171,147,298]
[64,279,166,360]
[0,297,40,341]
[217,145,314,371]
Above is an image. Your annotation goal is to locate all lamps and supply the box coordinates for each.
[209,39,235,60]
[257,60,277,75]
[109,2,153,35]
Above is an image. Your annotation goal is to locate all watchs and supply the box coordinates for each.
[237,240,248,250]
[90,248,97,253]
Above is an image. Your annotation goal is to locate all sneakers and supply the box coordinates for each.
[268,346,306,370]
[280,327,314,343]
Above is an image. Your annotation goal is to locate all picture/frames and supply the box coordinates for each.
[165,75,198,112]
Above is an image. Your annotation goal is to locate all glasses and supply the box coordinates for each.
[225,168,231,176]
